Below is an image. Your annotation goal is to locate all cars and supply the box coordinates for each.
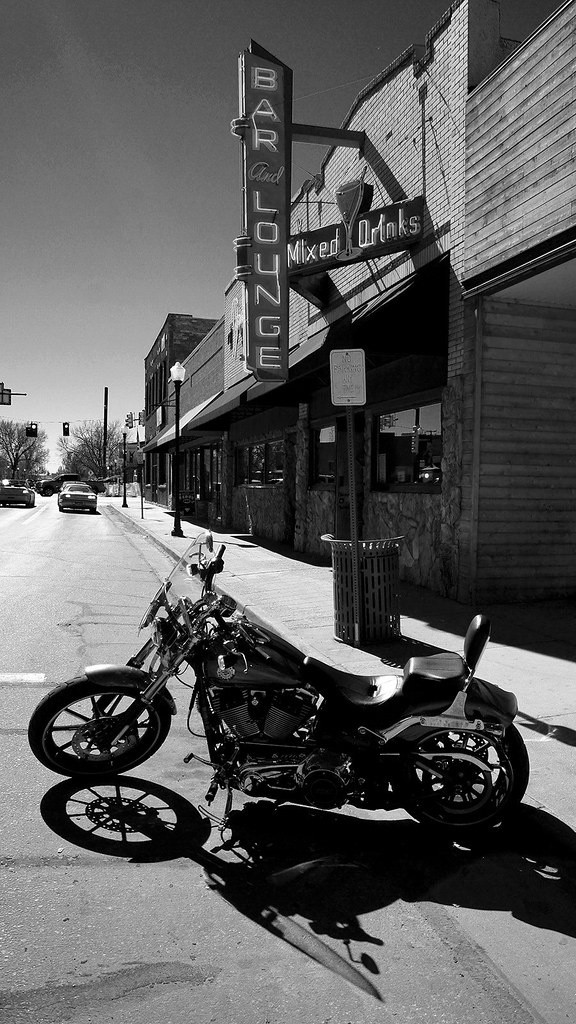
[0,479,35,508]
[58,481,97,513]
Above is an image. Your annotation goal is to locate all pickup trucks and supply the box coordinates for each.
[35,474,106,497]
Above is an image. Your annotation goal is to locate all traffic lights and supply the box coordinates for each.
[125,411,133,430]
[26,423,38,437]
[139,410,146,427]
[63,423,69,436]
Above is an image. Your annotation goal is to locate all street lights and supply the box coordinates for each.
[169,361,185,537]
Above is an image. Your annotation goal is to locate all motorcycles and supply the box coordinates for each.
[28,526,529,840]
